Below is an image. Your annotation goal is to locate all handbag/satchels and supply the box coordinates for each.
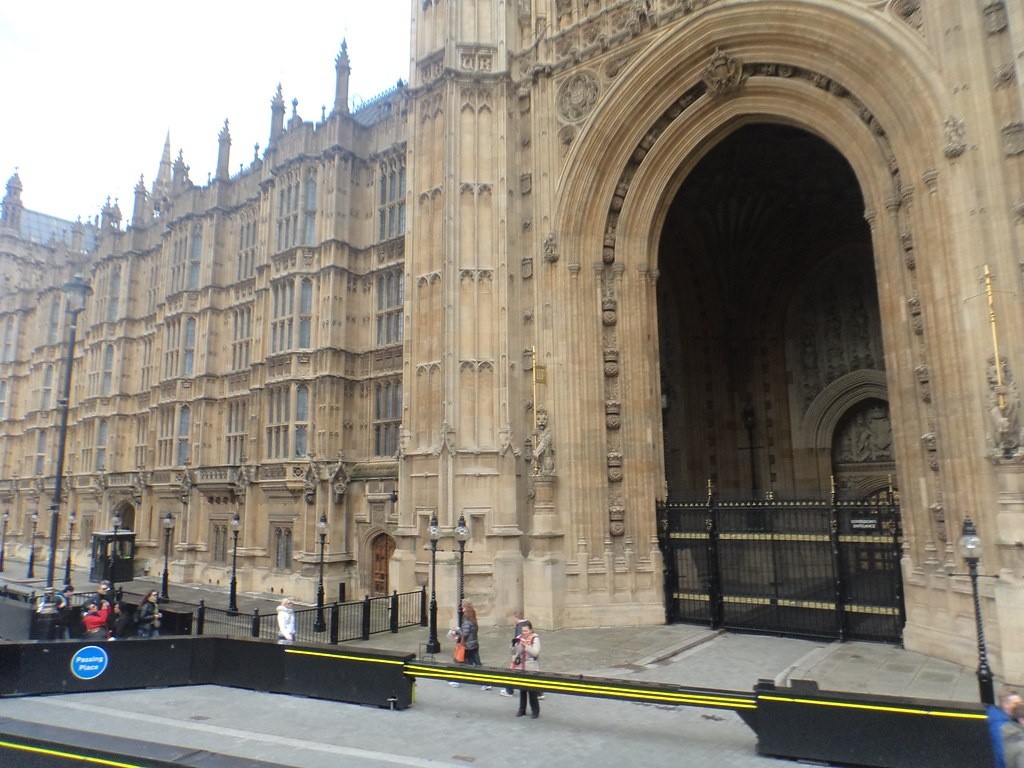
[454,633,466,663]
[154,603,161,628]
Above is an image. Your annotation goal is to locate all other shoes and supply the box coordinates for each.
[532,711,538,719]
[516,709,526,717]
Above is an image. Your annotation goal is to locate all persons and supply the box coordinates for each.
[57,580,162,640]
[449,599,544,719]
[276,597,296,641]
[987,695,1024,768]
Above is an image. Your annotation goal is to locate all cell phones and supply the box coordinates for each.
[514,638,520,642]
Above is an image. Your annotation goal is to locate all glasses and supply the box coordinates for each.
[103,588,107,590]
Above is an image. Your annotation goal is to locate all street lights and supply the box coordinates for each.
[450,513,474,644]
[958,511,996,707]
[44,273,97,587]
[224,512,244,616]
[62,510,77,592]
[313,510,330,633]
[25,511,40,579]
[108,509,124,601]
[0,507,11,573]
[160,511,177,605]
[422,511,445,653]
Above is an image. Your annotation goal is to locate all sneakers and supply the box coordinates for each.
[448,682,460,688]
[537,695,545,700]
[481,685,492,691]
[500,690,513,697]
